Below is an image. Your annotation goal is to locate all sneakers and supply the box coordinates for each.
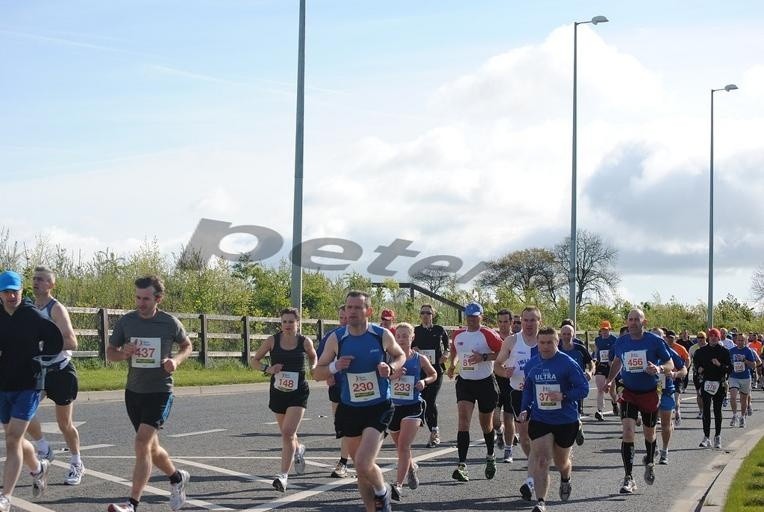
[642,445,669,485]
[108,501,135,512]
[723,399,728,407]
[273,473,287,492]
[430,431,440,444]
[708,328,720,337]
[595,411,604,422]
[452,464,469,482]
[484,429,518,479]
[714,435,722,448]
[426,435,435,448]
[374,463,419,512]
[697,413,703,419]
[170,470,190,512]
[575,421,584,446]
[675,417,681,425]
[1,492,12,512]
[295,445,305,474]
[331,461,348,478]
[620,475,637,493]
[612,401,618,415]
[699,437,711,448]
[31,447,86,498]
[730,405,753,427]
[520,477,571,512]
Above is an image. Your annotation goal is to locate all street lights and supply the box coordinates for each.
[569,13,612,335]
[703,83,740,328]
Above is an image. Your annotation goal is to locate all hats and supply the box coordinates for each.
[465,302,483,317]
[666,330,676,338]
[600,321,611,330]
[381,310,394,321]
[559,318,574,329]
[0,271,22,293]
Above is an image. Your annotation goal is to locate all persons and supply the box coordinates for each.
[0,271,63,512]
[25,265,86,486]
[106,274,193,512]
[251,290,763,511]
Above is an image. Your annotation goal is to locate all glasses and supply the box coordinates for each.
[513,321,521,325]
[420,311,432,314]
